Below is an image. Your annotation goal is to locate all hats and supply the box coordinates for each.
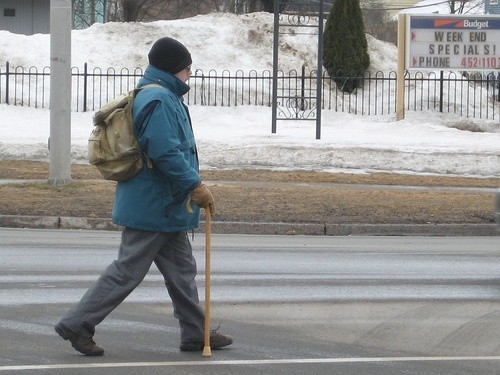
[148,37,192,74]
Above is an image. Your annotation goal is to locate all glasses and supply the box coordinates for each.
[187,64,191,70]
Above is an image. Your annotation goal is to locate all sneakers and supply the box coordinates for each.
[54,321,104,356]
[180,330,233,351]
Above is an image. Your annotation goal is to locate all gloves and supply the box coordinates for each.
[190,183,214,213]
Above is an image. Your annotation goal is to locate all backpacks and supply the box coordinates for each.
[88,84,165,181]
